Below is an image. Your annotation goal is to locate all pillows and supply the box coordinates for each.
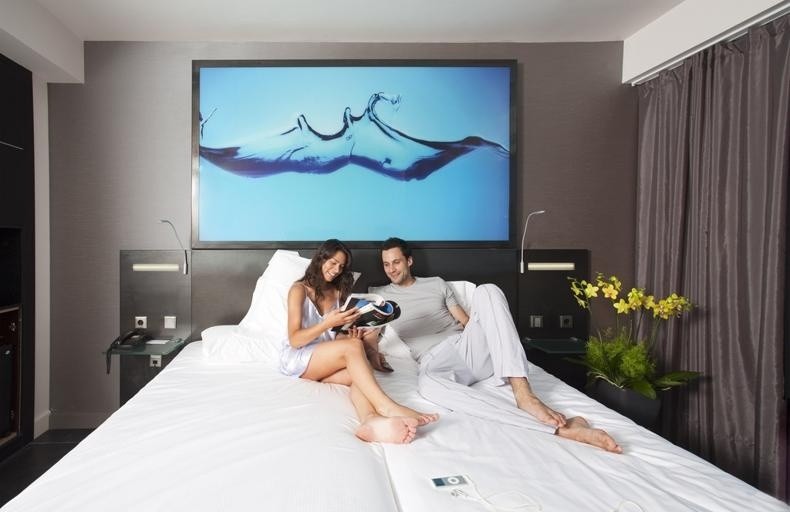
[201,325,280,365]
[235,247,364,336]
[368,281,479,367]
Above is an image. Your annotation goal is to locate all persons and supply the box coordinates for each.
[279,239,440,444]
[365,238,623,454]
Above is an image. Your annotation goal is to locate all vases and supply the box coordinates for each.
[583,377,673,430]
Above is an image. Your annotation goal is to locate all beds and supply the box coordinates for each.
[0,251,790,512]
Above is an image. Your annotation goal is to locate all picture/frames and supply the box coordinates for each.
[192,60,523,248]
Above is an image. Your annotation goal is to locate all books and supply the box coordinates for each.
[331,293,401,333]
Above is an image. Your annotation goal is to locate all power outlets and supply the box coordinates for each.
[150,354,163,368]
[135,316,147,328]
[560,315,574,329]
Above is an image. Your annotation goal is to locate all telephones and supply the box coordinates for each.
[106,330,149,354]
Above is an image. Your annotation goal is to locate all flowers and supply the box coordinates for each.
[565,272,704,398]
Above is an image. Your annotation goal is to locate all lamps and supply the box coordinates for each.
[157,219,189,277]
[518,210,546,274]
